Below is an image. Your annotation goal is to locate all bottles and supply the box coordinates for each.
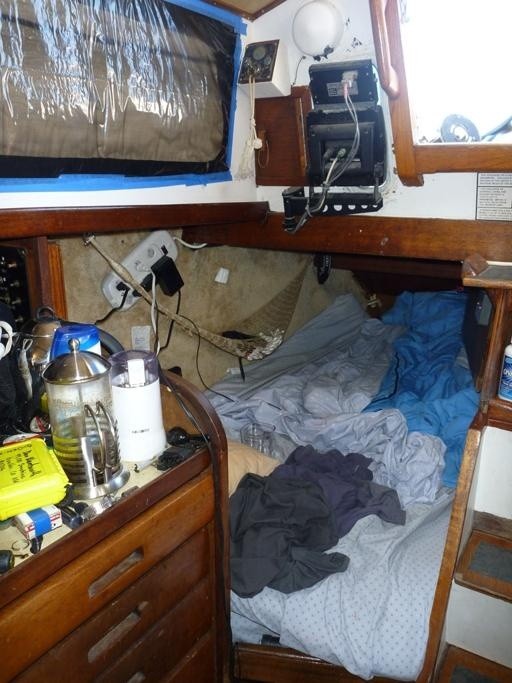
[108,347,168,462]
[41,336,131,499]
[497,337,512,404]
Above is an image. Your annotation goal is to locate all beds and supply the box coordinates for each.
[203,292,481,683]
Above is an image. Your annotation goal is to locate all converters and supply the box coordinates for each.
[149,257,185,297]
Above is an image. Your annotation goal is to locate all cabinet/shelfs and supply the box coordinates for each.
[1,368,231,683]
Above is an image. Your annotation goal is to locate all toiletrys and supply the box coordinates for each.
[49,325,101,358]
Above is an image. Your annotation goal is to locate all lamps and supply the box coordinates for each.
[293,0,344,61]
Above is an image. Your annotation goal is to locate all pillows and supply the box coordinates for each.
[228,438,281,497]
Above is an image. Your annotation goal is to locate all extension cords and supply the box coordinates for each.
[102,229,182,312]
